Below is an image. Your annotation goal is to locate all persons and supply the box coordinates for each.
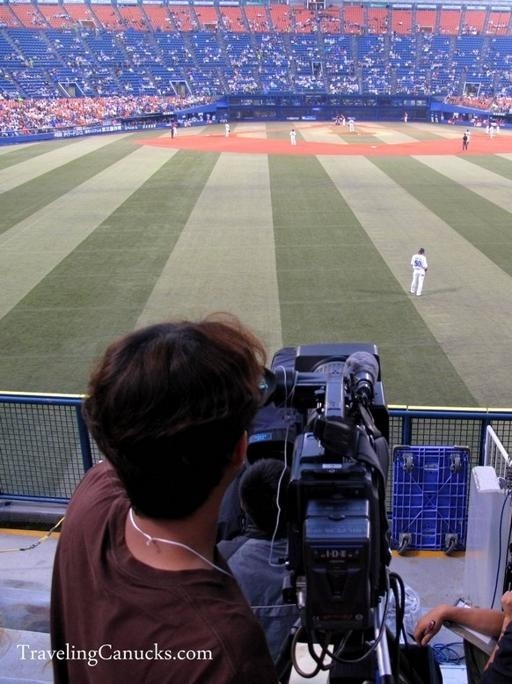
[0,5,512,150]
[414,591,512,684]
[409,248,428,296]
[50,311,276,684]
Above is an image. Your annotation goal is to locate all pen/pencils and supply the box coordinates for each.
[423,620,435,636]
